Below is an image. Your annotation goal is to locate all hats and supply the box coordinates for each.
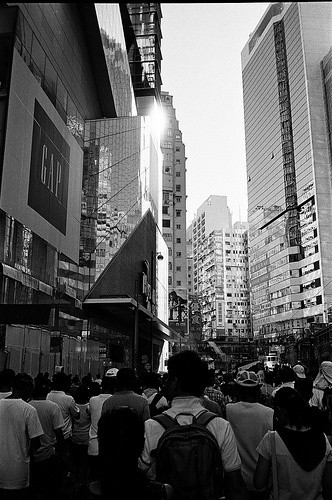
[292,365,307,378]
[105,368,119,377]
[234,371,258,387]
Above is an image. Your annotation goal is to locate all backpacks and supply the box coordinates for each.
[313,386,332,412]
[148,393,162,419]
[151,410,225,500]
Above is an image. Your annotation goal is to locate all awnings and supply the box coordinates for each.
[83,298,183,343]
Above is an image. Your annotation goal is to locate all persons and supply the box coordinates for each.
[0,350,332,500]
